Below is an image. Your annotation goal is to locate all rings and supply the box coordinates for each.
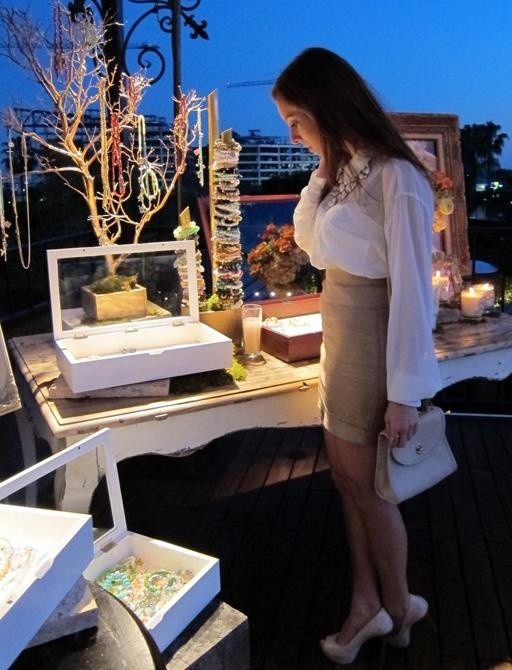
[389,434,399,441]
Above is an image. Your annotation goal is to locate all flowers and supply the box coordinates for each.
[432,171,455,232]
[246,222,309,293]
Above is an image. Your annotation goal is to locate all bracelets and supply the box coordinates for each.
[172,137,246,313]
[93,553,193,626]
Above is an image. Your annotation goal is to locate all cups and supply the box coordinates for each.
[240,303,264,364]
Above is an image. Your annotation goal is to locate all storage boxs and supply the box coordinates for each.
[0,420,248,661]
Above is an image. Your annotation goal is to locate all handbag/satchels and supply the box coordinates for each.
[373,407,459,507]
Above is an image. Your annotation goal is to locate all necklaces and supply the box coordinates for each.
[0,77,208,270]
[53,5,66,77]
[326,161,371,209]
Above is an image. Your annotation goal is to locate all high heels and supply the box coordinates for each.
[379,593,439,649]
[321,606,395,667]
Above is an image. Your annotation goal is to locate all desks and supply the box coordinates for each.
[6,306,512,511]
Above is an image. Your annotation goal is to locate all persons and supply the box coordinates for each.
[267,44,444,668]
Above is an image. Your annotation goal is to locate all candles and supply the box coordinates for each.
[431,270,495,322]
